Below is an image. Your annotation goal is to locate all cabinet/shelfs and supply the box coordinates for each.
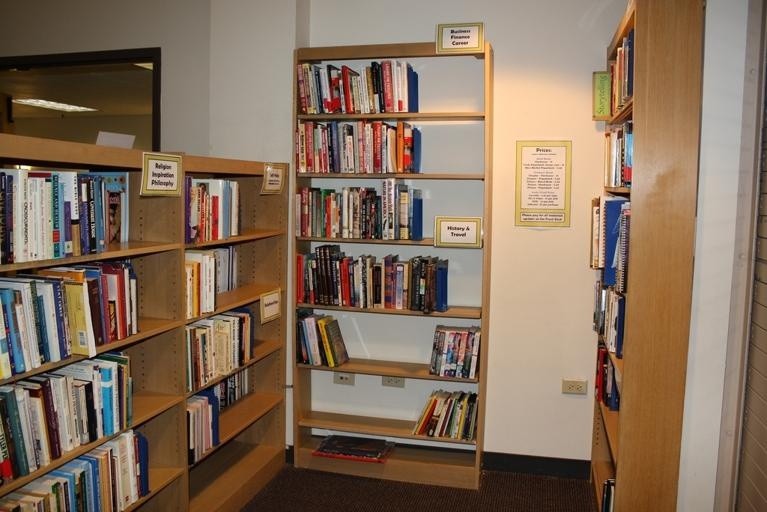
[291,40,495,493]
[0,130,291,512]
[586,1,706,512]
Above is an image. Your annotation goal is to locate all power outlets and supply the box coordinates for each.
[562,378,587,395]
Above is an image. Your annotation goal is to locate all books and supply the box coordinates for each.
[186,311,252,393]
[295,119,420,173]
[430,325,481,379]
[185,246,239,319]
[1,170,129,264]
[185,177,241,243]
[296,59,418,115]
[296,177,424,240]
[297,309,349,367]
[186,368,249,465]
[312,435,395,463]
[589,29,634,512]
[0,259,137,379]
[296,245,448,313]
[1,351,149,512]
[413,390,479,441]
